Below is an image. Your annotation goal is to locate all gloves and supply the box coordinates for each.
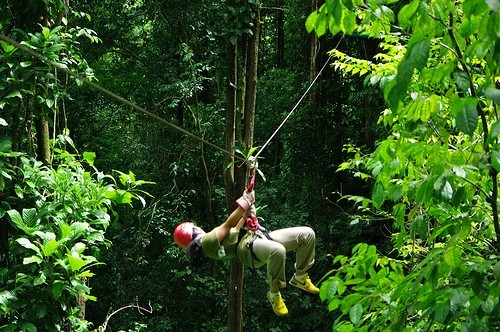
[235,188,256,211]
[243,204,256,220]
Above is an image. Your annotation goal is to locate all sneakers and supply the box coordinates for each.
[289,272,321,294]
[266,289,289,316]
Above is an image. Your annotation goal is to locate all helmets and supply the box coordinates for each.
[173,221,194,251]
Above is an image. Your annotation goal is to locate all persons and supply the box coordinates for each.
[174,188,322,316]
